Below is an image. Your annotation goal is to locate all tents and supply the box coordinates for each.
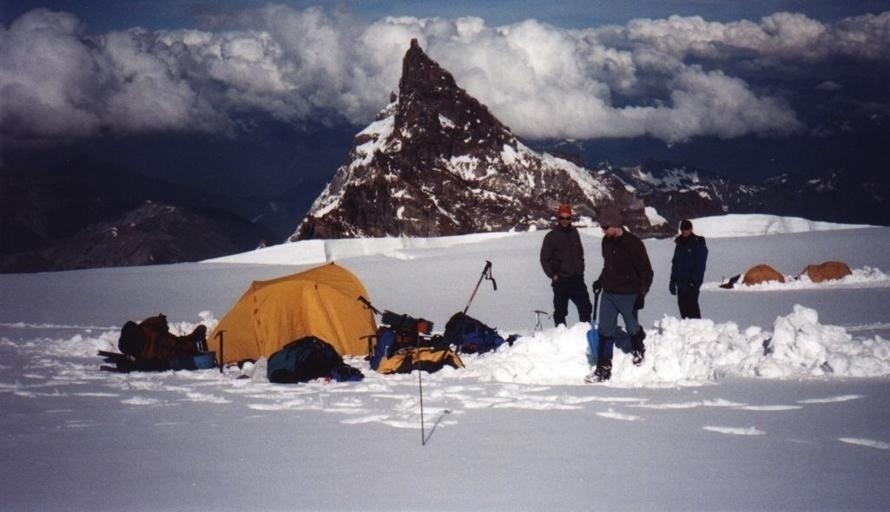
[204,259,381,367]
[739,263,786,287]
[796,260,852,284]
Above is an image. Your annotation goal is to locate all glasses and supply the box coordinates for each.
[601,225,609,230]
[558,216,571,220]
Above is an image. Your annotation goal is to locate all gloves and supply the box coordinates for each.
[633,295,645,310]
[669,280,676,295]
[593,280,602,294]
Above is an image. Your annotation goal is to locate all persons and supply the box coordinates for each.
[538,203,593,327]
[667,217,709,320]
[583,205,655,385]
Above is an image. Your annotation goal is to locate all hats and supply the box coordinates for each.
[555,204,575,218]
[598,207,623,228]
[680,220,692,231]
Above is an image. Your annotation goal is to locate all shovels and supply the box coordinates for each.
[587,285,602,359]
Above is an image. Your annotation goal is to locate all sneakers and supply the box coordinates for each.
[632,346,645,364]
[584,366,611,383]
